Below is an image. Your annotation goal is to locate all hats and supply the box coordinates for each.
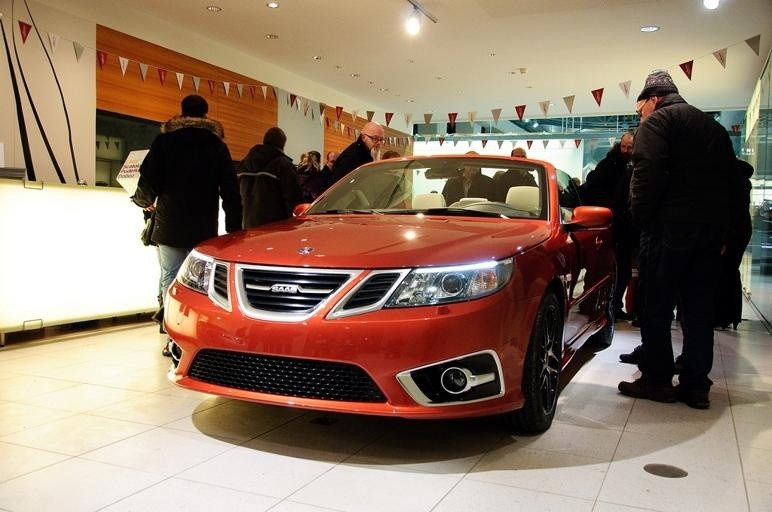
[637,69,679,102]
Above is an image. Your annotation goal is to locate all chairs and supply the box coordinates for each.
[507,187,540,214]
[412,193,446,208]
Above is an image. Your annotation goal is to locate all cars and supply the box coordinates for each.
[163,154,621,434]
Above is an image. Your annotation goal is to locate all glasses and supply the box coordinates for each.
[636,99,648,117]
[364,134,385,144]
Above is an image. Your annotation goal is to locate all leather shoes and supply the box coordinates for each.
[619,346,710,409]
[616,309,641,327]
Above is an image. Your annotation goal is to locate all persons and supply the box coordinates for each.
[569,132,753,332]
[441,151,494,206]
[238,127,303,229]
[132,95,244,356]
[498,147,539,202]
[333,121,384,185]
[382,149,400,160]
[320,153,338,189]
[143,209,166,333]
[618,72,743,408]
